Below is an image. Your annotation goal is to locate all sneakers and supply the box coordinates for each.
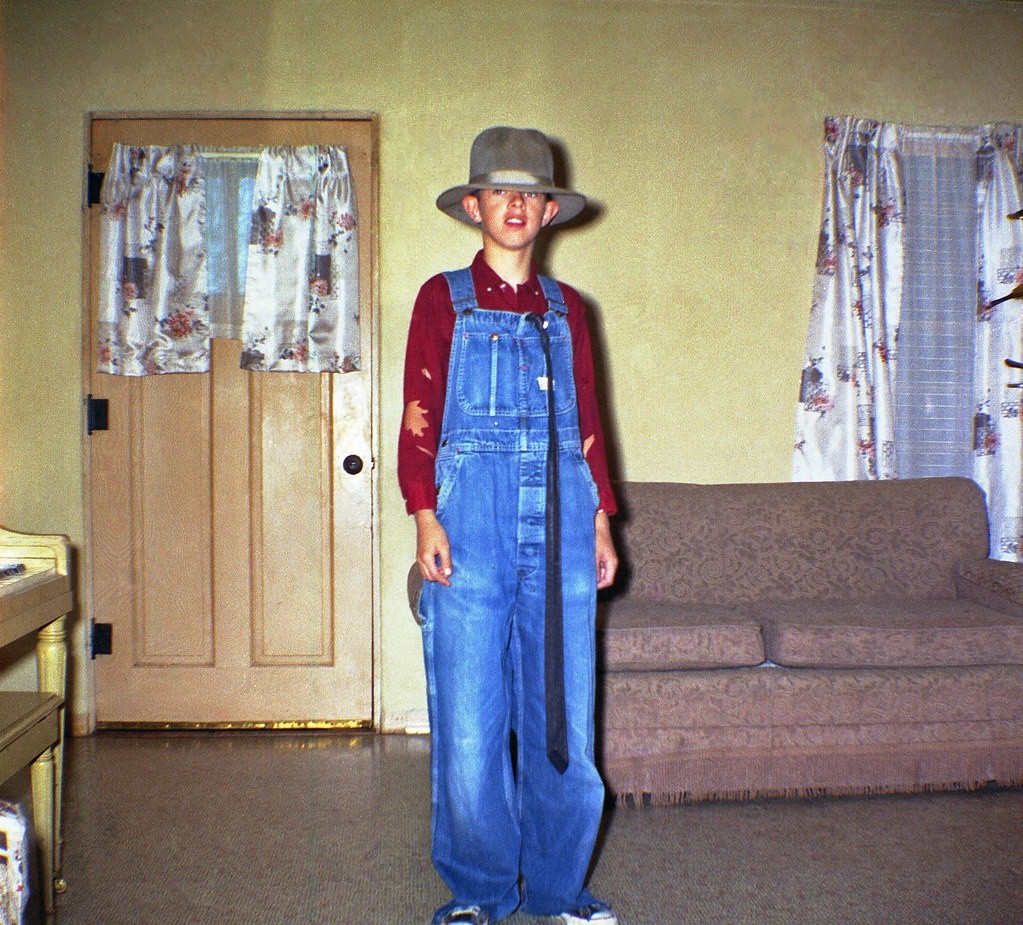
[441,904,491,924]
[556,900,619,924]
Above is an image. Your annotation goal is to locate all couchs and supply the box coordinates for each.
[558,476,1021,808]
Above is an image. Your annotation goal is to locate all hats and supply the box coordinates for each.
[436,127,586,230]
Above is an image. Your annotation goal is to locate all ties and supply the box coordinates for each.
[525,310,571,775]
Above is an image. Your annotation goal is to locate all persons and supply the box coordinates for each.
[400,128,619,925]
